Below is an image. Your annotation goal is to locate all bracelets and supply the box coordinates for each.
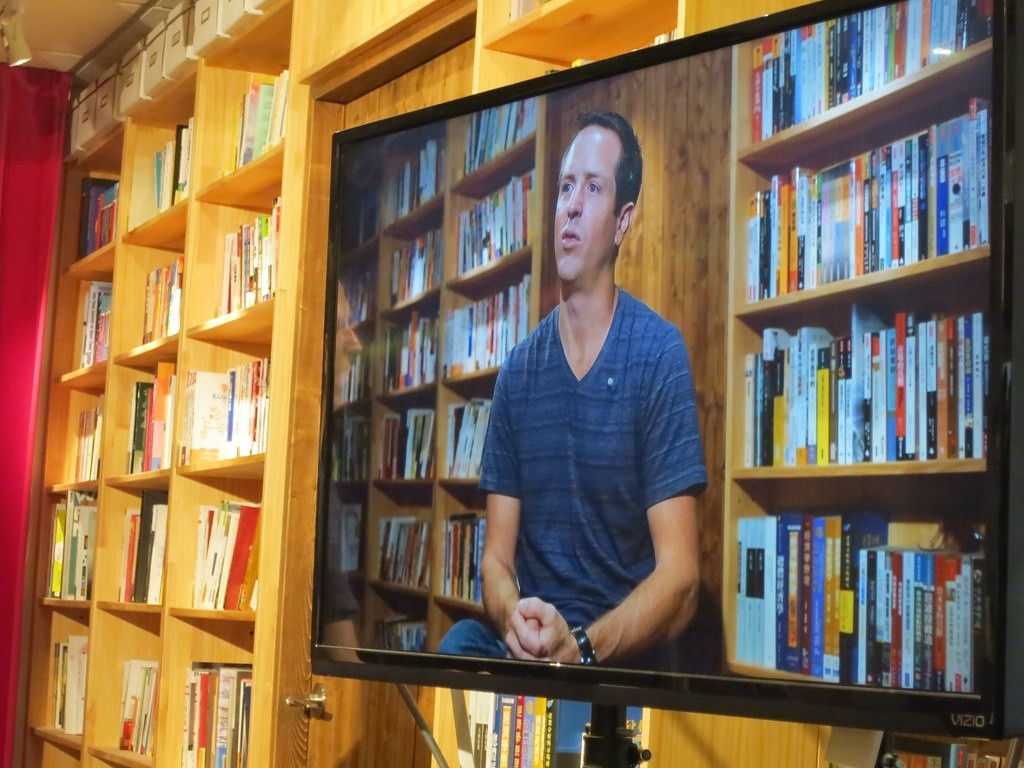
[572,626,597,665]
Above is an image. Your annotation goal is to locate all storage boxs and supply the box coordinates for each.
[69,0,264,155]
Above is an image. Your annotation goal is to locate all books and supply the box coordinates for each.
[233,69,289,170]
[738,0,988,690]
[142,257,185,345]
[154,117,194,210]
[193,500,261,611]
[117,490,170,605]
[221,197,281,315]
[335,97,537,652]
[877,742,1002,768]
[71,395,105,483]
[80,281,111,370]
[47,490,96,600]
[180,355,269,466]
[50,637,87,736]
[78,178,120,257]
[182,661,252,767]
[126,375,176,474]
[115,661,159,758]
[467,691,643,768]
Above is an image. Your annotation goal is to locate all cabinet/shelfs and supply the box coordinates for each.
[720,0,991,694]
[10,0,292,768]
[322,92,561,657]
[432,0,852,768]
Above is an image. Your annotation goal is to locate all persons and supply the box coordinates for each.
[438,111,708,670]
